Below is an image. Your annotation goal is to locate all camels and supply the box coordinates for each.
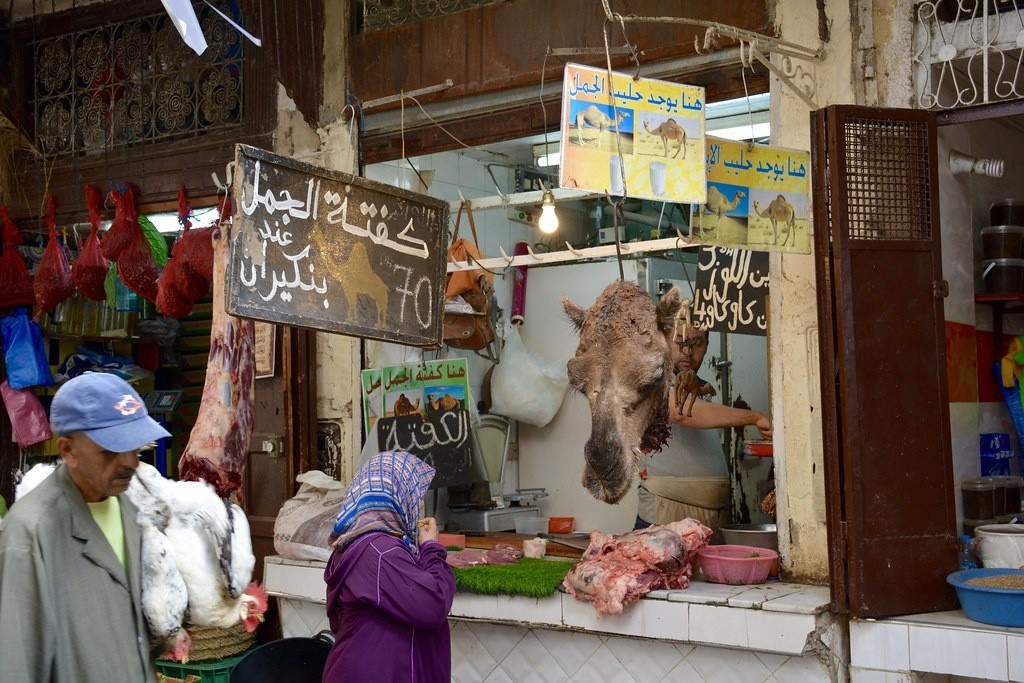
[427,393,461,411]
[576,105,630,148]
[754,195,796,246]
[698,186,746,238]
[560,277,685,505]
[394,393,419,416]
[643,117,687,160]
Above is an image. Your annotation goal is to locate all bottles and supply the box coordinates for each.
[958,535,977,570]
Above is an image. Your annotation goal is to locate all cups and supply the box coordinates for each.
[515,517,548,536]
[549,517,574,533]
[649,162,666,195]
[610,156,627,193]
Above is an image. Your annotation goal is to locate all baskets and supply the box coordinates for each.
[698,545,778,585]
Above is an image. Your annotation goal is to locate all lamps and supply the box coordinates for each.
[531,110,770,167]
[949,149,1005,178]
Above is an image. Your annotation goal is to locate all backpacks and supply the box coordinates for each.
[442,200,501,365]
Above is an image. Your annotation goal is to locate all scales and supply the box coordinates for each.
[448,411,548,535]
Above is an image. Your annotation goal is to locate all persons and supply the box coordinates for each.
[323,451,458,683]
[632,306,774,545]
[0,371,173,683]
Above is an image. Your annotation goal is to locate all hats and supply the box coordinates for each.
[50,371,173,452]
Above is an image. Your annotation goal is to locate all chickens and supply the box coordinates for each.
[11,456,271,668]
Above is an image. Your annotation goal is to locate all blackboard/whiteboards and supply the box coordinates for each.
[225,144,450,350]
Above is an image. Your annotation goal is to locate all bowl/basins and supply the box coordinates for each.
[988,198,1024,226]
[946,568,1024,628]
[719,524,777,551]
[980,226,1024,260]
[982,258,1024,293]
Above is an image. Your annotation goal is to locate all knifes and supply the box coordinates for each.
[537,533,586,550]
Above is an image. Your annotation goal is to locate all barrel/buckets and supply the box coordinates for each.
[975,524,1024,569]
[229,630,336,683]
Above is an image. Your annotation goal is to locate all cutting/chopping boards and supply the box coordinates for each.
[439,530,591,559]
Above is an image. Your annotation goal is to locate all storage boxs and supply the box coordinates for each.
[153,646,260,683]
